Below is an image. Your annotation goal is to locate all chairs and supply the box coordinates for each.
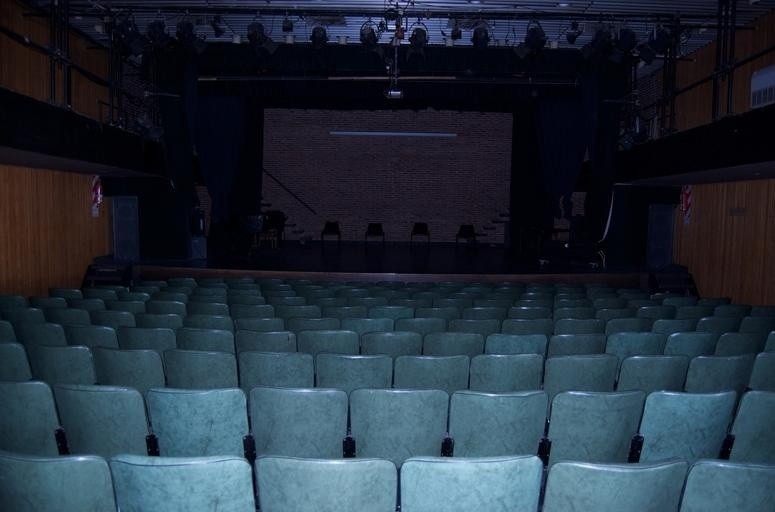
[109,453,257,511]
[546,390,646,463]
[393,354,469,389]
[0,278,775,352]
[748,352,775,391]
[635,390,741,461]
[617,353,690,391]
[0,454,116,511]
[411,223,430,242]
[543,353,619,391]
[316,352,392,389]
[163,349,238,387]
[248,386,349,458]
[456,225,475,243]
[321,221,342,241]
[92,346,163,386]
[542,457,688,512]
[145,386,250,456]
[684,353,756,391]
[53,383,154,455]
[448,389,549,457]
[349,389,450,457]
[238,352,314,387]
[1,380,63,455]
[470,354,543,389]
[679,459,775,512]
[400,454,543,512]
[255,455,398,512]
[365,223,385,242]
[727,391,775,464]
[0,343,33,381]
[257,211,283,248]
[29,345,96,384]
